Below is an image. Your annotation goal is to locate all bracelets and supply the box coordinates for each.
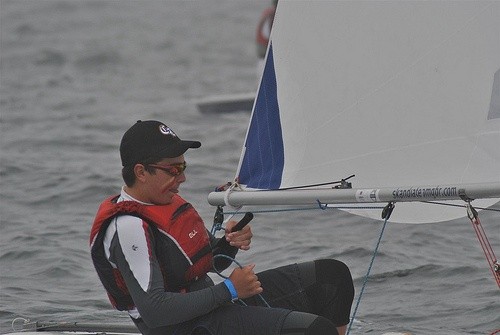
[224,279,237,301]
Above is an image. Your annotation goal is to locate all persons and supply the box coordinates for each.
[92,119,354,335]
[255,0,278,90]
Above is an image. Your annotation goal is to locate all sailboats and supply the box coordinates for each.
[1,0,500,335]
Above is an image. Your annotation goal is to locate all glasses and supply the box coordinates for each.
[137,161,186,176]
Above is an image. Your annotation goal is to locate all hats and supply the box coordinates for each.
[120,120,201,166]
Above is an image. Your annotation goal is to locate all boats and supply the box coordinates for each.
[197,92,260,115]
